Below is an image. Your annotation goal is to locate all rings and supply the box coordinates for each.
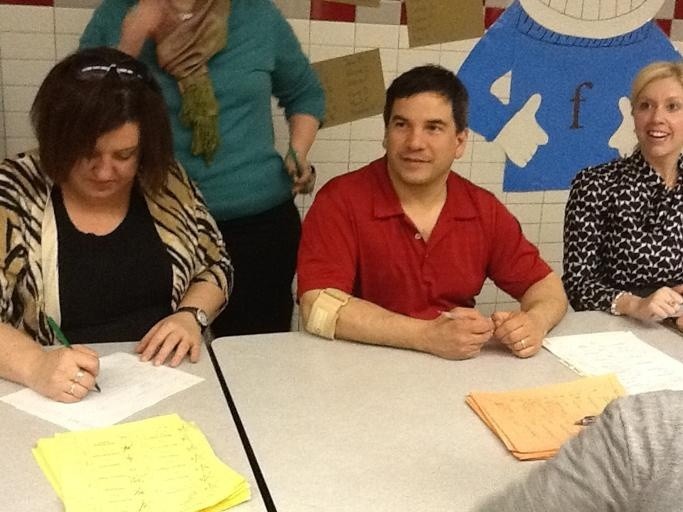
[68,382,76,396]
[520,338,527,349]
[310,165,316,175]
[74,368,83,383]
[670,299,677,306]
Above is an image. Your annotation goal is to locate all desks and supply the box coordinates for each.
[211,309,682,512]
[2,341,268,512]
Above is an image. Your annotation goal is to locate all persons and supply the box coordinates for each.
[0,47,237,403]
[561,60,682,335]
[78,1,327,335]
[296,63,571,359]
[470,390,683,512]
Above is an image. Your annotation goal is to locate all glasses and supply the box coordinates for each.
[74,64,146,86]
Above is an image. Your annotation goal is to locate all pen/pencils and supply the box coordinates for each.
[438,310,474,320]
[574,416,595,425]
[46,316,101,394]
[288,141,313,199]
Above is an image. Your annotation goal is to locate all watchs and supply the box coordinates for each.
[176,305,210,334]
